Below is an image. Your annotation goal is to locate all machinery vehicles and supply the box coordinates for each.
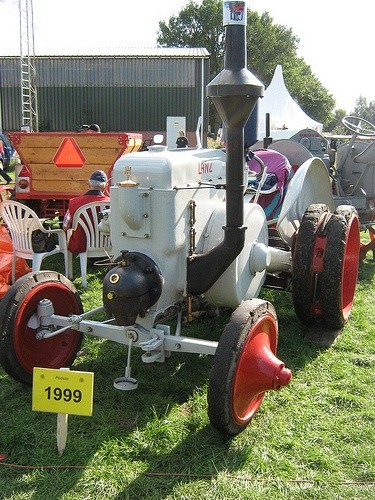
[0,1,375,439]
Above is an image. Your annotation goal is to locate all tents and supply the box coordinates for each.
[220,65,323,148]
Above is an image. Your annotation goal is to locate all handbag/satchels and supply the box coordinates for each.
[30,224,57,253]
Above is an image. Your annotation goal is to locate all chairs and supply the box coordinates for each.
[247,149,291,218]
[0,200,69,283]
[66,200,111,288]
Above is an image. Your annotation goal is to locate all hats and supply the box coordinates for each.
[78,125,90,131]
[90,170,108,183]
[85,124,100,131]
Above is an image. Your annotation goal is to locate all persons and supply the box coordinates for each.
[176,130,188,148]
[78,125,90,133]
[38,170,111,267]
[85,124,101,134]
